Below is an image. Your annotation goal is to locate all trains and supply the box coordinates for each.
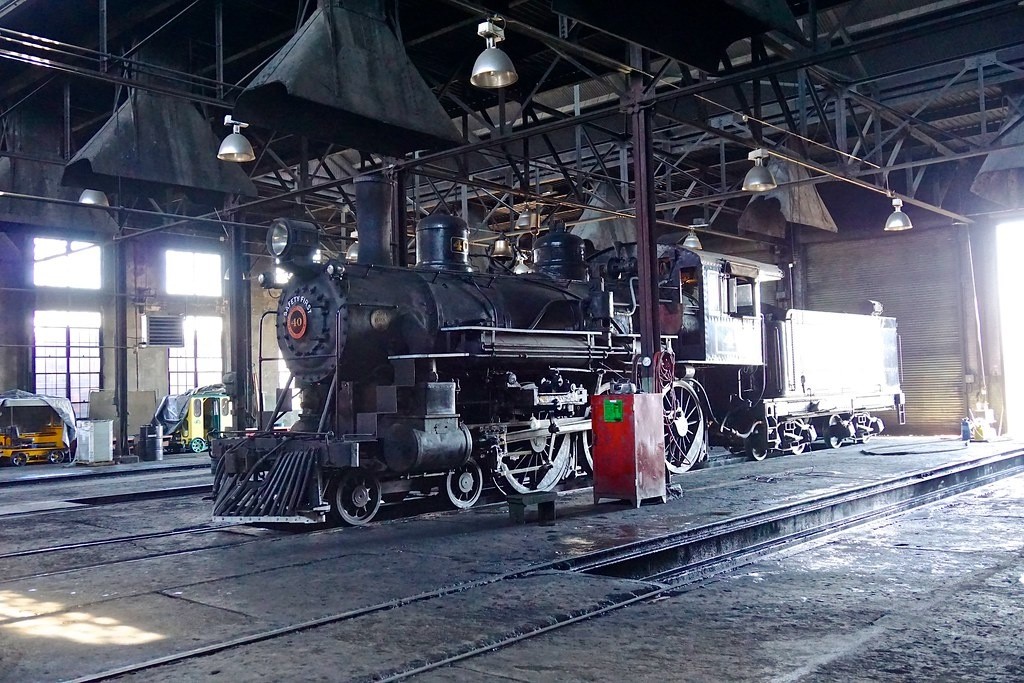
[200,208,907,528]
